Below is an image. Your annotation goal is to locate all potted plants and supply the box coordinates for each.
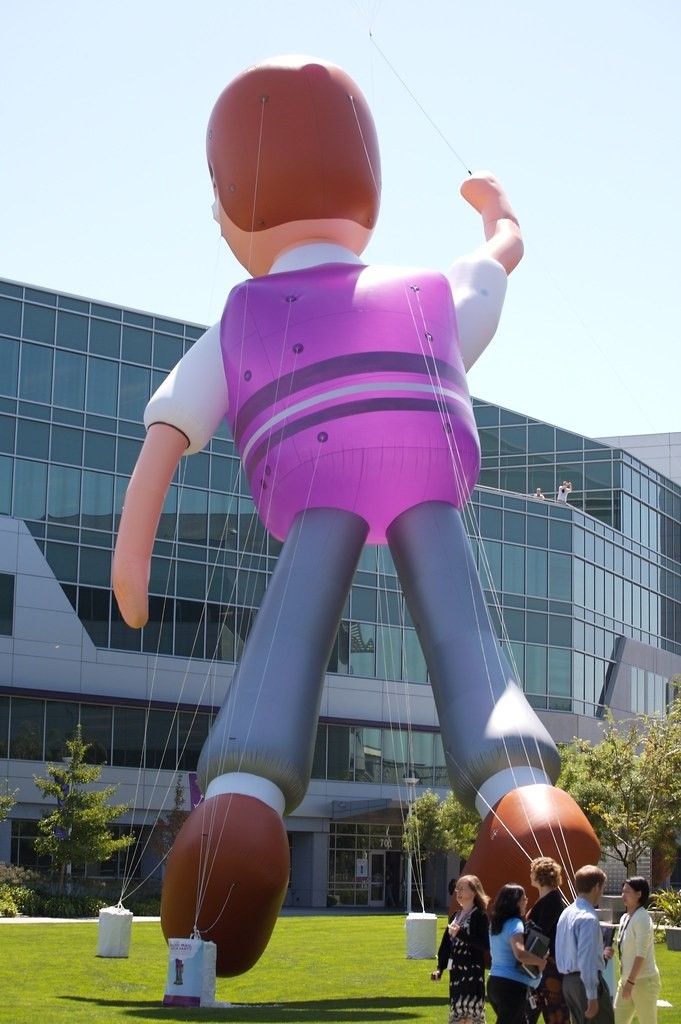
[645,884,681,953]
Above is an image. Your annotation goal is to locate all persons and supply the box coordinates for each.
[557,481,572,502]
[431,857,661,1024]
[534,488,545,498]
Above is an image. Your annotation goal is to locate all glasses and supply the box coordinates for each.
[454,886,470,892]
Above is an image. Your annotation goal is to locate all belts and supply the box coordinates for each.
[570,971,581,976]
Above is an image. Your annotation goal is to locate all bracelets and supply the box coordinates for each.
[627,980,635,985]
